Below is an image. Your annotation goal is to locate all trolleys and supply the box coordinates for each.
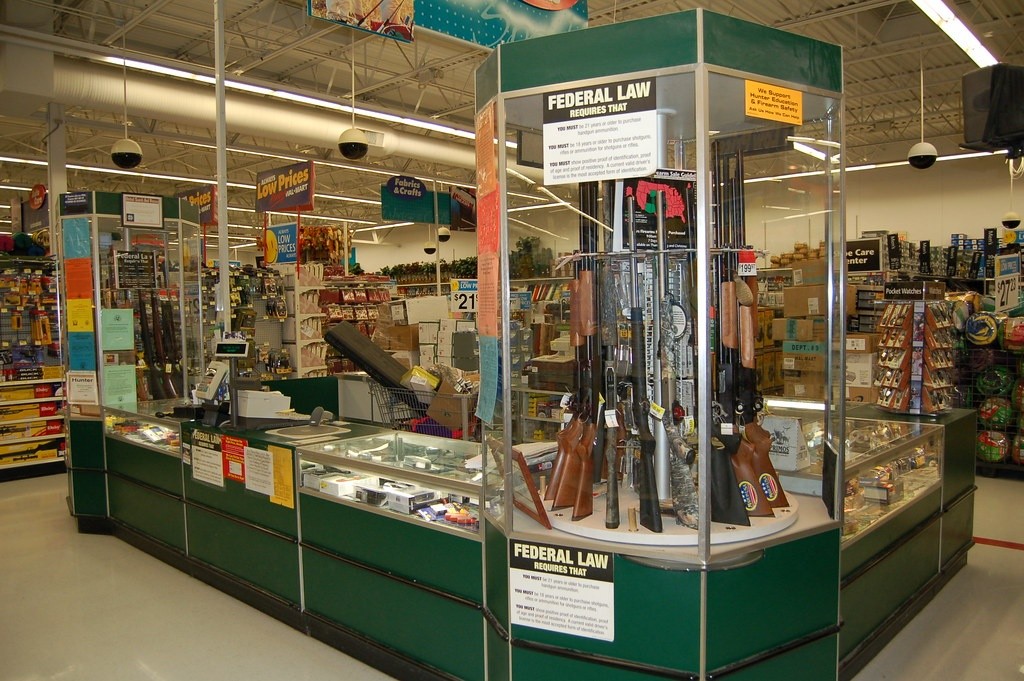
[364,376,480,443]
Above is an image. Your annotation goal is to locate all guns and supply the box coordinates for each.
[541,136,792,534]
[106,233,193,403]
[0,424,51,436]
[0,441,59,460]
[0,406,41,415]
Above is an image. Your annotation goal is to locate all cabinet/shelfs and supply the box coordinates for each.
[0,257,68,482]
[511,388,568,444]
[397,268,792,306]
[323,280,393,375]
[282,271,327,378]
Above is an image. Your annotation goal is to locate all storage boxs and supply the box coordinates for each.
[0,420,66,440]
[0,401,66,421]
[304,473,379,497]
[372,296,533,385]
[0,440,66,465]
[0,382,66,402]
[772,259,883,402]
[427,380,475,430]
[400,366,440,390]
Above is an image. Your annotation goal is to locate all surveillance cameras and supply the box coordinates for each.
[424,242,437,254]
[438,228,451,242]
[111,139,143,169]
[1002,212,1020,229]
[338,128,368,160]
[908,142,937,170]
